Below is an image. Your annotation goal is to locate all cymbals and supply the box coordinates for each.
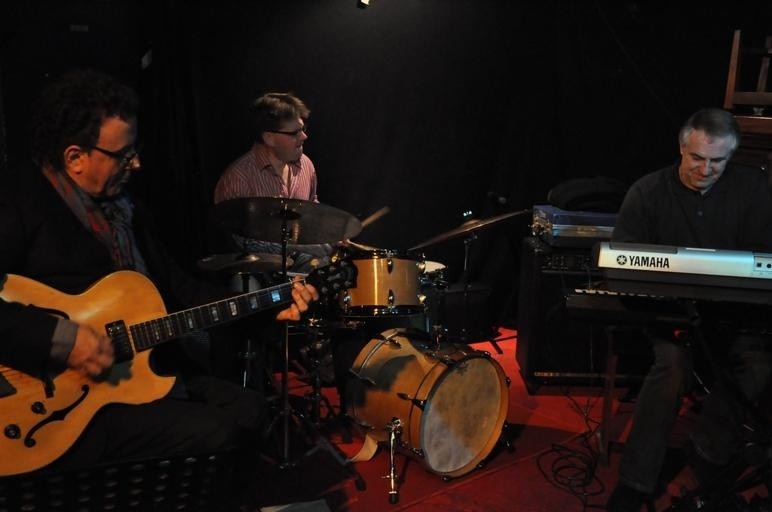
[205,253,293,269]
[406,208,535,249]
[205,196,363,244]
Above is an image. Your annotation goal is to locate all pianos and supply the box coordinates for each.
[565,242,771,321]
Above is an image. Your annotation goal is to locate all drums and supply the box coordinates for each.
[348,328,510,481]
[301,250,448,338]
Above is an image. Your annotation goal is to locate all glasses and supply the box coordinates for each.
[266,125,307,139]
[93,146,139,170]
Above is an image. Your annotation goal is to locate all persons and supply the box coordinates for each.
[602,107,772,512]
[211,91,321,268]
[0,65,321,512]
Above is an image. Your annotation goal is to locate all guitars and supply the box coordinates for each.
[0,259,357,476]
